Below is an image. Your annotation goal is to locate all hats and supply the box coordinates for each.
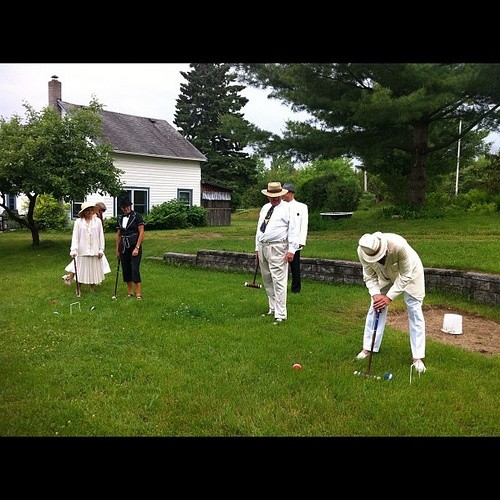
[117,196,132,208]
[261,182,290,198]
[358,231,388,264]
[77,202,101,218]
[282,182,296,193]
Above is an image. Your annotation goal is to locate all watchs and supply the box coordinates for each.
[135,246,140,250]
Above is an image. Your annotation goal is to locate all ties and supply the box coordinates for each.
[259,205,275,233]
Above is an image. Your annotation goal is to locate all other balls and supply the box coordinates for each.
[90,306,97,311]
[384,373,392,381]
[292,363,302,372]
[53,311,61,316]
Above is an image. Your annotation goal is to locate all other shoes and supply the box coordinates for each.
[272,318,285,325]
[355,349,371,360]
[412,359,426,372]
[125,293,141,302]
[261,312,274,317]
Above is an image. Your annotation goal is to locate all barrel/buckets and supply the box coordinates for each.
[440,313,463,334]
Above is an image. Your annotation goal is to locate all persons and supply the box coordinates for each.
[255,181,296,325]
[354,232,426,373]
[62,202,111,298]
[116,197,145,302]
[280,183,309,293]
